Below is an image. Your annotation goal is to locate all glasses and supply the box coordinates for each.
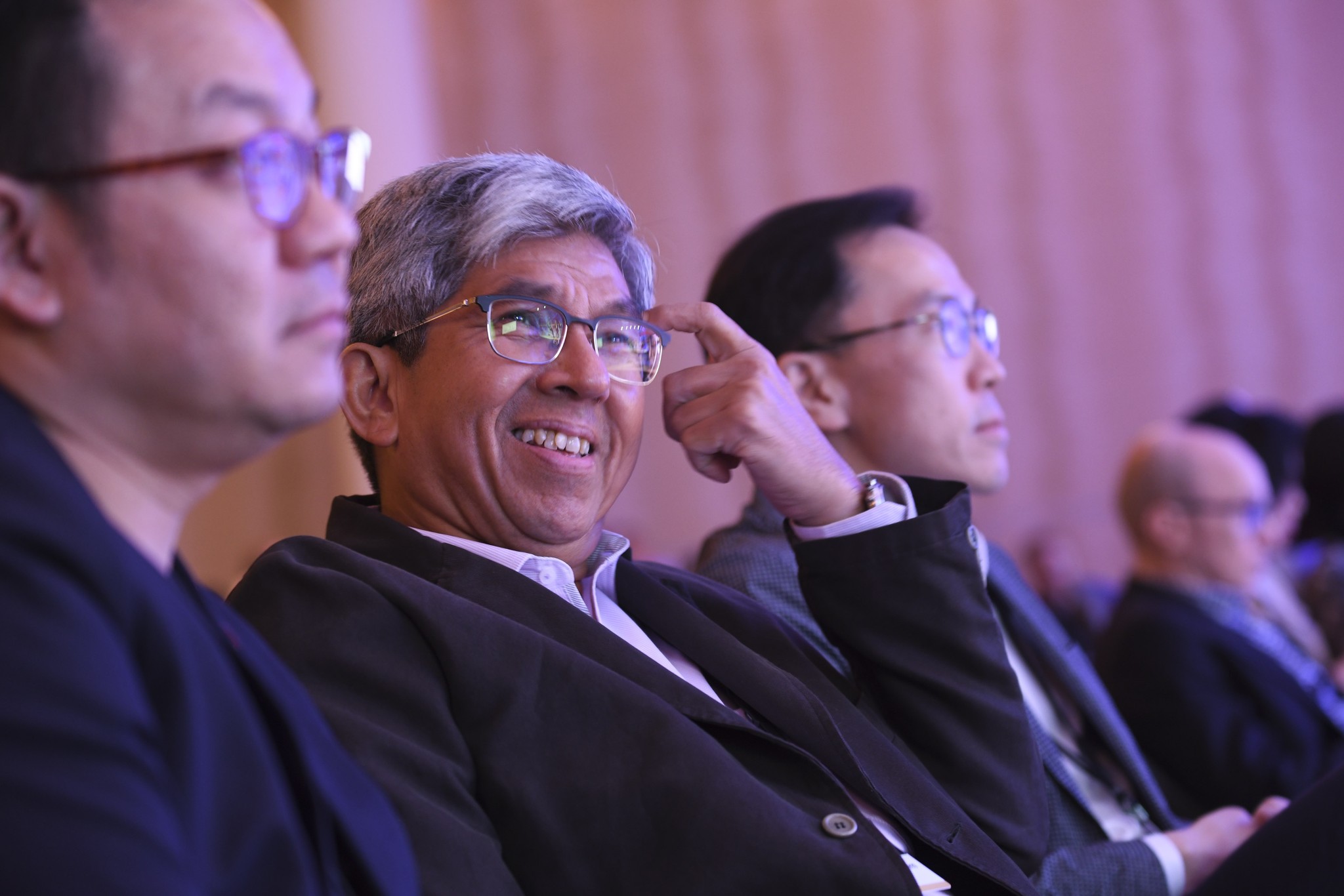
[374,293,670,385]
[1189,495,1268,525]
[800,298,1001,359]
[15,125,372,227]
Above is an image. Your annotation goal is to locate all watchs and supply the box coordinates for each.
[866,478,900,510]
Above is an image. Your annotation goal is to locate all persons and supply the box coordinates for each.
[703,186,1344,896]
[226,153,1053,896]
[1095,401,1344,896]
[0,0,422,896]
